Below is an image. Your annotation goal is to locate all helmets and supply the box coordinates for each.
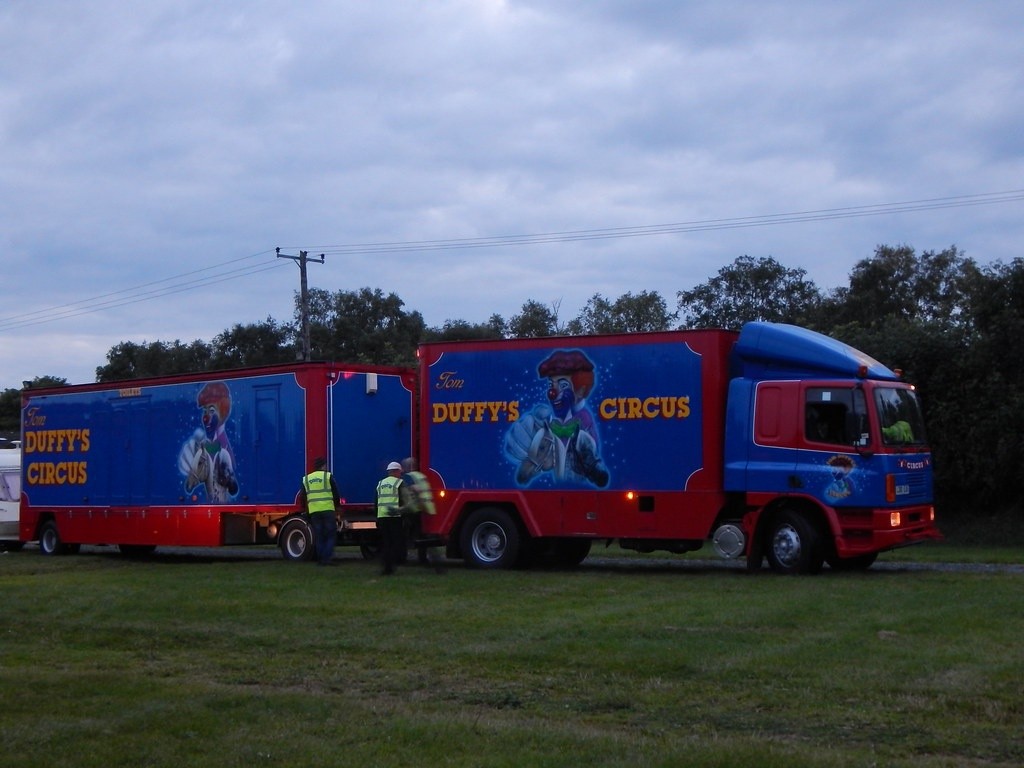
[386,461,403,471]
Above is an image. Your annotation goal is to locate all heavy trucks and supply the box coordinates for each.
[19,310,939,575]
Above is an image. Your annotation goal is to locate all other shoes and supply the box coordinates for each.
[379,569,395,574]
[317,560,338,566]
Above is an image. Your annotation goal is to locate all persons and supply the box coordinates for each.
[373,461,412,575]
[300,457,340,564]
[389,456,437,567]
